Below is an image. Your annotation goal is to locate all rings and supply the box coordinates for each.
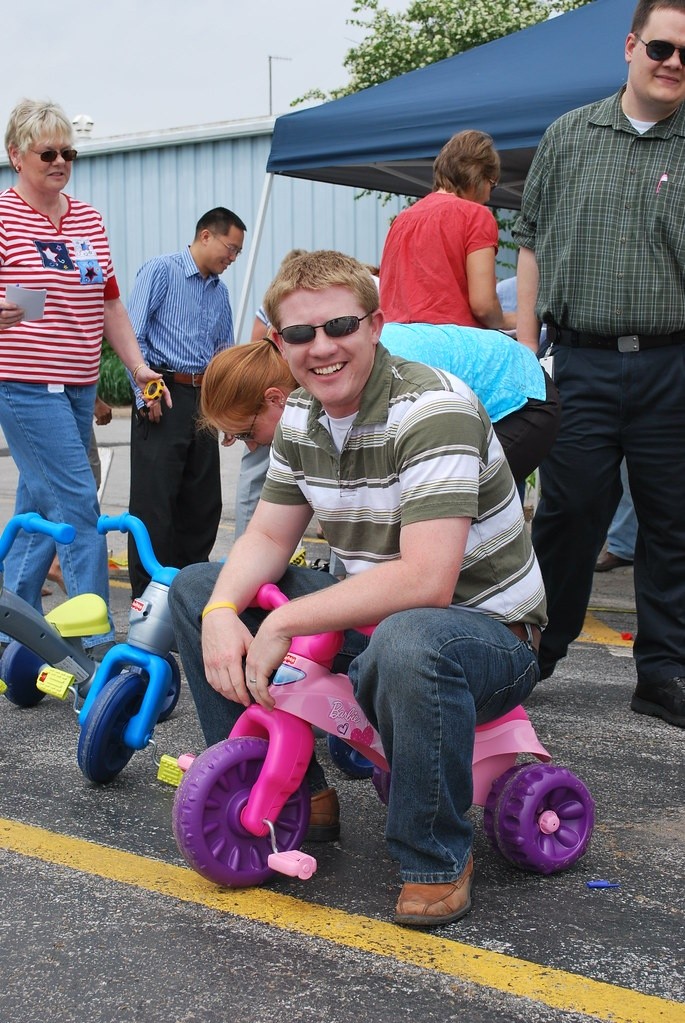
[250,678,256,682]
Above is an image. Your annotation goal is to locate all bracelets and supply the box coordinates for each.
[200,602,238,619]
[133,364,146,379]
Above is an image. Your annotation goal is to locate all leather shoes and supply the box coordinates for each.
[393,850,475,925]
[306,788,343,842]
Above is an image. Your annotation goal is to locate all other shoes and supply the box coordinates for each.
[535,653,554,682]
[85,641,115,664]
[629,675,685,727]
[595,553,634,572]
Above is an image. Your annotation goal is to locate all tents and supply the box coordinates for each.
[235,0,645,341]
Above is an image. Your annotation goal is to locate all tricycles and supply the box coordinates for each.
[0,511,181,723]
[35,511,188,790]
[170,584,595,887]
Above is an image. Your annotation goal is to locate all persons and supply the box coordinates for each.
[166,250,549,925]
[515,0,685,728]
[592,455,640,571]
[127,207,247,604]
[378,129,519,334]
[230,251,382,560]
[494,275,517,317]
[0,100,172,669]
[38,395,112,597]
[197,323,562,514]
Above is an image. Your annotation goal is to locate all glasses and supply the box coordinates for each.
[635,34,685,67]
[277,309,374,344]
[487,177,498,191]
[26,147,78,162]
[232,412,260,441]
[211,233,242,258]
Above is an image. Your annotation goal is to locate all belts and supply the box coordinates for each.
[506,622,541,653]
[545,325,685,353]
[156,369,204,388]
